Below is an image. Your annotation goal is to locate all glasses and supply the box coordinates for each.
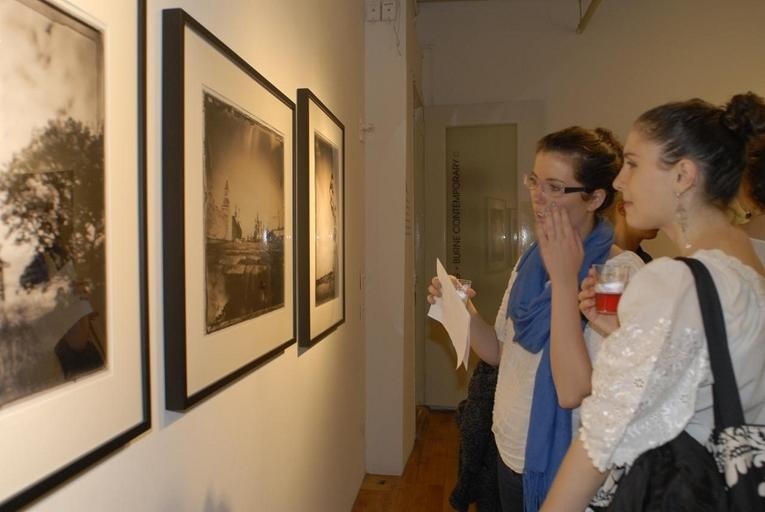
[522,173,591,197]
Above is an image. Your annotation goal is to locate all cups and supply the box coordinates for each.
[593,263,630,314]
[450,279,470,308]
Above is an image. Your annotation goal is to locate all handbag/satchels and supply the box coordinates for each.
[592,423,765,512]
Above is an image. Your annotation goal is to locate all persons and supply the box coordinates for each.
[538,89,765,511]
[608,194,658,263]
[425,122,647,511]
